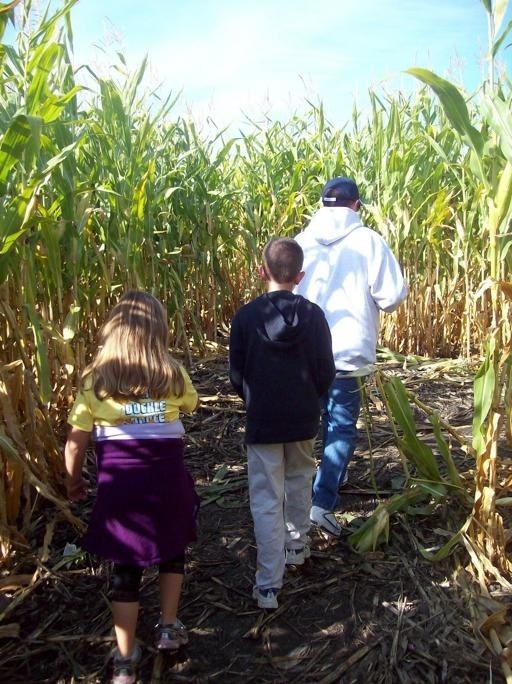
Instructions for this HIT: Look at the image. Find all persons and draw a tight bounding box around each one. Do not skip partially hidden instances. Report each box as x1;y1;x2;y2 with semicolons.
283;174;407;536
64;288;199;683
226;234;335;609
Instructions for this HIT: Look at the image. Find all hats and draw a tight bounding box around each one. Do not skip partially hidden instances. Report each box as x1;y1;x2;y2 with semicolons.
322;177;359;201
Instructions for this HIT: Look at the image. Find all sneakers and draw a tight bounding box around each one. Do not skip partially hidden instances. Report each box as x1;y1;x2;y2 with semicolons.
112;647;142;684
155;620;188;649
253;585;278;608
285;545;310;564
310;506;341;536
